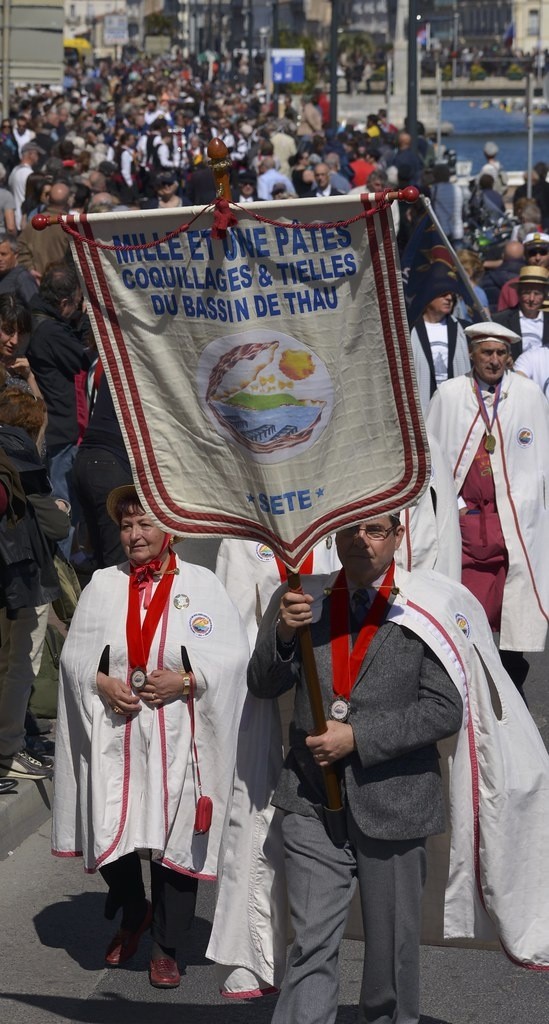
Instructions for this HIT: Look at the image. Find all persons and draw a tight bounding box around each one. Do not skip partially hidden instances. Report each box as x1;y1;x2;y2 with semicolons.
0;30;549;1023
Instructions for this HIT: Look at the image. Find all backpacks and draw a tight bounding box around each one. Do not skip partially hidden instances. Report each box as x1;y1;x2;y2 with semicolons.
27;622;67;719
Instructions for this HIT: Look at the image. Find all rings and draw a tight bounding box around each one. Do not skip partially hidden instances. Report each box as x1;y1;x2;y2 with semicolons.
113;709;119;714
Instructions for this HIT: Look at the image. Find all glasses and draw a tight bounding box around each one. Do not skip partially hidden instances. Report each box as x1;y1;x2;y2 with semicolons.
340;522;399;542
524;249;549;256
162;181;176;187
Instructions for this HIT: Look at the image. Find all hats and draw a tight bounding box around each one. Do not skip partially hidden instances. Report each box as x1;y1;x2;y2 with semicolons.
509;265;549;290
464;321;521;348
21;143;47;155
106;483;185;546
523;232;549;250
160;172;176;183
484;141;498;156
271;183;286;194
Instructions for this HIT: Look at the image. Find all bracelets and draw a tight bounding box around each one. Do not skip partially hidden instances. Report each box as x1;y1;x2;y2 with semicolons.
181;672;190;695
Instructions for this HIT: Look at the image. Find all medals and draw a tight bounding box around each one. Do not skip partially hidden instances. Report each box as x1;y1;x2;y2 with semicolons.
328;697;351;723
130;668;148;691
485;434;496;451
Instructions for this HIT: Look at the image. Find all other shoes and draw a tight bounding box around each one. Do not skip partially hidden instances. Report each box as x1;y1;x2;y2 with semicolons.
25;738;56;755
19;741;53;767
151;941;180;987
0;776;19;794
105;899;153;964
0;751;54;778
23;718;52;735
